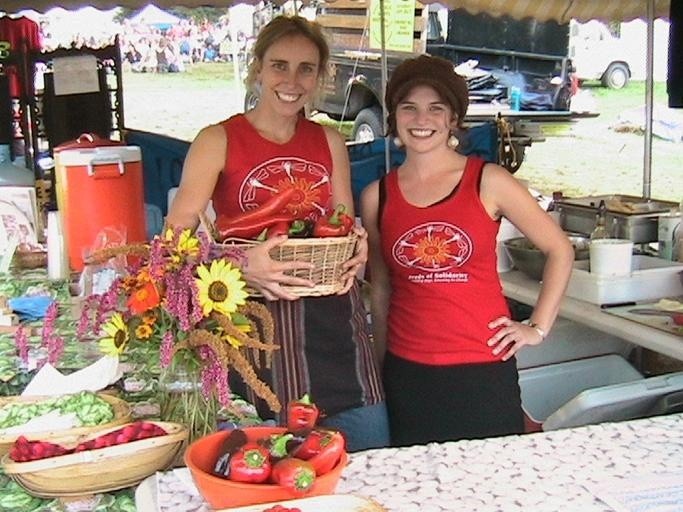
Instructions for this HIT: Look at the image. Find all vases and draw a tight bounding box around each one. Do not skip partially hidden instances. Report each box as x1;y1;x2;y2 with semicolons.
140;345;223;473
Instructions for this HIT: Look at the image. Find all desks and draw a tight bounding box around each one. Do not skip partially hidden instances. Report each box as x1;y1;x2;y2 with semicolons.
131;407;681;512
493;256;682;364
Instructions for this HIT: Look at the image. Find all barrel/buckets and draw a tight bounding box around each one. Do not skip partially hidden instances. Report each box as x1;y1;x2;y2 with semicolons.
146;205;164;237
53;134;145;272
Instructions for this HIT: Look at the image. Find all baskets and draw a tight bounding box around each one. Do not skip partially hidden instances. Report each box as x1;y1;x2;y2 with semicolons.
15;252;47;267
216;232;357;297
0;394;188;498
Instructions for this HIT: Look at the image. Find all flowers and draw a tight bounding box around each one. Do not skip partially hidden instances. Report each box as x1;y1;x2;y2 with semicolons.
6;220;288;468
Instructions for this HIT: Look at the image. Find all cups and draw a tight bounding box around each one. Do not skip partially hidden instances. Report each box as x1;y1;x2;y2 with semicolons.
587;238;634;280
657;214;680;260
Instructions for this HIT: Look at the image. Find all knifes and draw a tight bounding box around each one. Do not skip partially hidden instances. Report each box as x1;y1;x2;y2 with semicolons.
595;296;659;310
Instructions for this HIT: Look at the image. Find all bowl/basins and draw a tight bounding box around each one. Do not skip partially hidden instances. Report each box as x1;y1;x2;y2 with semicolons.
182;426;348;511
502;237;590;282
12;251;47;269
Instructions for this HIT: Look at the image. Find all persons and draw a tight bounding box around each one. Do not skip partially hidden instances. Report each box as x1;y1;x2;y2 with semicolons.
159;16;391;453
358;54;575;448
37;16;258;76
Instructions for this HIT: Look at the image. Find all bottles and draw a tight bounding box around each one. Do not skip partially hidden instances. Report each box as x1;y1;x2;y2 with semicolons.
510;84;520;112
588;199;612;270
546;191;563;214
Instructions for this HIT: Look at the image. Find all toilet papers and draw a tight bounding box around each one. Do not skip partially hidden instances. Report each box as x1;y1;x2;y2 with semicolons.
48;211;71;281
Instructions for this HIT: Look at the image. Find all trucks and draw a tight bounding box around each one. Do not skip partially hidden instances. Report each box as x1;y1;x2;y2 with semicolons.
241;0;602;176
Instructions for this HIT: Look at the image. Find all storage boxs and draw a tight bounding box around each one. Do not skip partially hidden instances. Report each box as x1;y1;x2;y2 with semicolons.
563;254;682;308
514;316;683;435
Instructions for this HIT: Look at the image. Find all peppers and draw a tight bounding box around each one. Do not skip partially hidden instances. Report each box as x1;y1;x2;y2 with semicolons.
212;187;353;242
229;394;344;491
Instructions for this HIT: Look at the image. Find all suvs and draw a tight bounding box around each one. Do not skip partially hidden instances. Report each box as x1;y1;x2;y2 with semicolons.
565;16;638;92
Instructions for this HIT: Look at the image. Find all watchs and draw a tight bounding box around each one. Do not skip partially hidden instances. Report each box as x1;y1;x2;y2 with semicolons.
520;320;546;340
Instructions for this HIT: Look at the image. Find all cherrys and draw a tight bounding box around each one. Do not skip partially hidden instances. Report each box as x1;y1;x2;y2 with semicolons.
9;422;166;462
